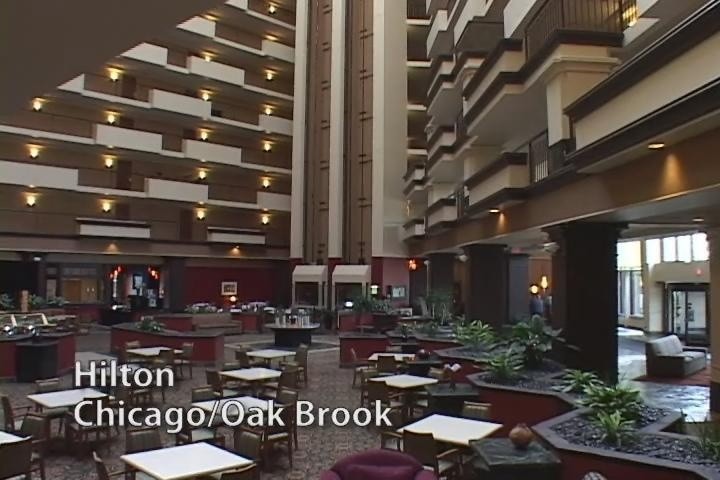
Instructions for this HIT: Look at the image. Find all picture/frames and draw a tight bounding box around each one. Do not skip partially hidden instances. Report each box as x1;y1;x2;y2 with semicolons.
388;285;408;300
218;279;240;297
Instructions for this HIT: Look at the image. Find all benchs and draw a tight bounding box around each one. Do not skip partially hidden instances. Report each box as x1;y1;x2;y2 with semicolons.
644;334;707;378
192;311;243;336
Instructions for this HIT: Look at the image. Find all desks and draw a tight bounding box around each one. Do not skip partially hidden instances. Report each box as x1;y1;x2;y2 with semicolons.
397;412;505;464
263;322;321;346
